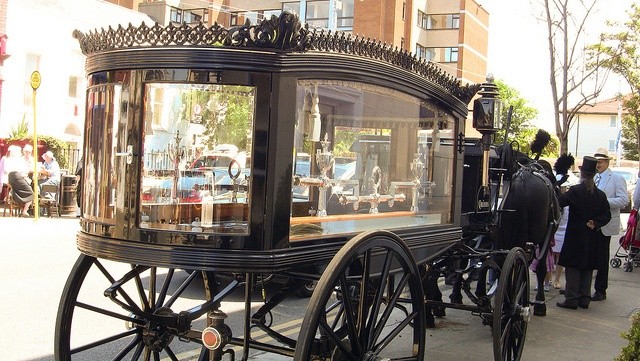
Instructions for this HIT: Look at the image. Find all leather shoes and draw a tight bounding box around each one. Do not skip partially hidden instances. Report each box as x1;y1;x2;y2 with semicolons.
591;290;606;301
579;301;588;309
557;300;578;309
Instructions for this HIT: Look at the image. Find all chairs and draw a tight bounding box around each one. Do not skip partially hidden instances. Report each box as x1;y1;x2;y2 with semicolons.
42;185;58;218
3;185;18;217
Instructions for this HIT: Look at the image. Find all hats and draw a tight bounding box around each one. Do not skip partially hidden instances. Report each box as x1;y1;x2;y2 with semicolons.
593;147;613;160
578;156;597;174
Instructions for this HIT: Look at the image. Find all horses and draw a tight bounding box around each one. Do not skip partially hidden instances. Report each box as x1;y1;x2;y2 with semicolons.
419;145;562;327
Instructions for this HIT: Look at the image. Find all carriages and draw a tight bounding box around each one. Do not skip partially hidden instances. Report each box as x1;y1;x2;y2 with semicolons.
54;9;570;360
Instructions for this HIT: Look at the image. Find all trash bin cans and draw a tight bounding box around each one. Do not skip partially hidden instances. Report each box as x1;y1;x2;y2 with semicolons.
61;175;78;213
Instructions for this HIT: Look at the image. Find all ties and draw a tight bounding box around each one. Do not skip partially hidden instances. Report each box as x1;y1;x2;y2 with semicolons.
595;173;600;187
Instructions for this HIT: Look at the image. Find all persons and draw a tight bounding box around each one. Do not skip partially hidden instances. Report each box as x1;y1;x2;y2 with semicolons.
37;151;61;216
632;170;640;210
0;145;15;203
189;147;202;167
590;147;630;300
21;145;34;185
529;234;557;292
549;174;570;289
74;157;83;217
555;156;612;310
7;146;35;218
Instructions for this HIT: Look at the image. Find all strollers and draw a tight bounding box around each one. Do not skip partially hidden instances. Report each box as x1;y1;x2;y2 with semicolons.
610;204;639;272
